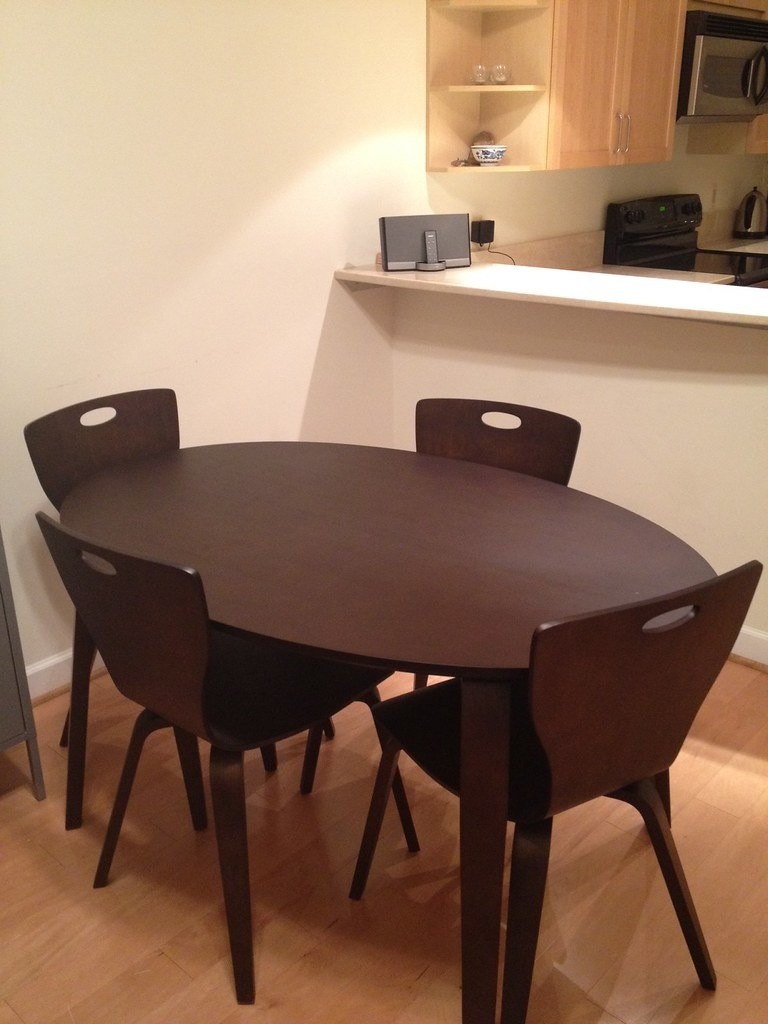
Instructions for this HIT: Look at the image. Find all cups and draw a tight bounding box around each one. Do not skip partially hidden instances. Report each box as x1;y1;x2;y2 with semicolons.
469;63;489;85
490;63;511;85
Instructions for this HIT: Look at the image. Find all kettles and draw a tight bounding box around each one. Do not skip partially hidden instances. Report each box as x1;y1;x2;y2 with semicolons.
733;186;768;239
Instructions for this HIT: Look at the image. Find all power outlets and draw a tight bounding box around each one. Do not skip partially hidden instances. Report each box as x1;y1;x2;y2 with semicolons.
472;214;490;251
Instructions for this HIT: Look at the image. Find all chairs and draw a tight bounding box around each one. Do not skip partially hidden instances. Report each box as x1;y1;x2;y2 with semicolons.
414;398;583;487
34;511;422;1006
347;559;766;1024
25;388;337;834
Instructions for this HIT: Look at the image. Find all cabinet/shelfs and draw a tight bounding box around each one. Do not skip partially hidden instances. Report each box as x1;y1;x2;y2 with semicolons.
685;114;768;154
426;0;688;174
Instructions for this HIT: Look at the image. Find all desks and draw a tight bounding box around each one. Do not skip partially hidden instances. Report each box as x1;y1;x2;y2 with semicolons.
573;264;736;285
698;236;768;274
60;441;719;1024
331;259;768;673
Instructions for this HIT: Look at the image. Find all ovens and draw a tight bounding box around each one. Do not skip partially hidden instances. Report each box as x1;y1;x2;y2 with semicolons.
676;10;768;127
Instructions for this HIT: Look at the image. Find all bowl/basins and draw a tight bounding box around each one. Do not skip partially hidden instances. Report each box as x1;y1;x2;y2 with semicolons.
470;145;507;167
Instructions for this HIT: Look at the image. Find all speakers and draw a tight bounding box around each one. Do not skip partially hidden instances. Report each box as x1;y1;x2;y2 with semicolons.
379;212;472;270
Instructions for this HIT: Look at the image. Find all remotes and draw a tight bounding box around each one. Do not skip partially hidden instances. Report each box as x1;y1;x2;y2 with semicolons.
424;230;438;264
